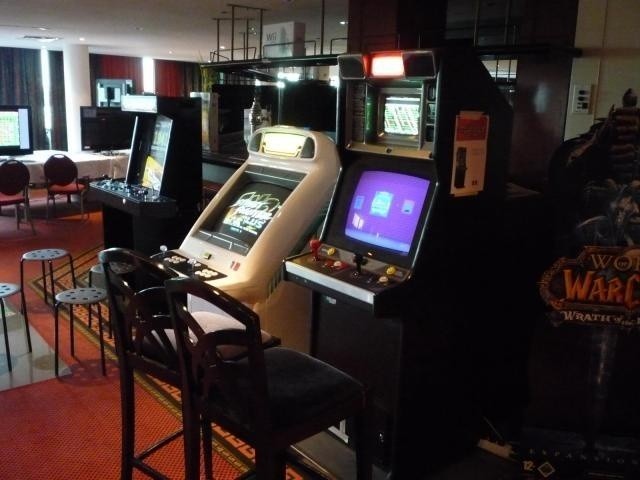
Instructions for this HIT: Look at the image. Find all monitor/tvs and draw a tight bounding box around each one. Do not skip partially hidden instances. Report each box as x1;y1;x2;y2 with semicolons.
96;79;134;106
208;179;298;247
121;95;159;115
0;105;34;157
378;93;421;138
344;168;431;260
79;105;137;151
139;118;176;190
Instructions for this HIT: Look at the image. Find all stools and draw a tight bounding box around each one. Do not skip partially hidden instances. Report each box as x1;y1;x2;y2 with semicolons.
19;246;78;325
0;283;33;375
86;264;115;341
50;288;110;377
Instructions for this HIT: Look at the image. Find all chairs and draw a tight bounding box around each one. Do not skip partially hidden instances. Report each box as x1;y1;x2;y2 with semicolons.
43;154;90;223
166;276;375;479
0;161;37;235
97;247;282;480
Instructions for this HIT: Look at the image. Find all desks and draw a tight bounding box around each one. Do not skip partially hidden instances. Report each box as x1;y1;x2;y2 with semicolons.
98;148;131;180
0;144;112;211
